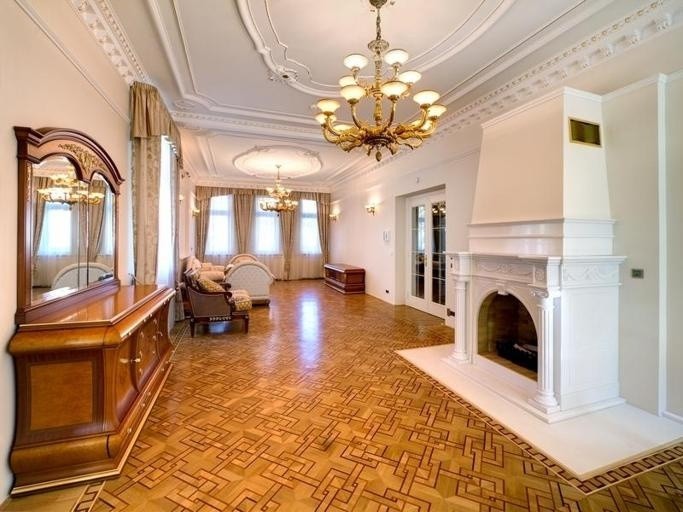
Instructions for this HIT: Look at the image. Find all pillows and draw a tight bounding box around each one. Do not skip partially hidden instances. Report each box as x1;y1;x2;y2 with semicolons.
194;277;226;294
200;262;211;270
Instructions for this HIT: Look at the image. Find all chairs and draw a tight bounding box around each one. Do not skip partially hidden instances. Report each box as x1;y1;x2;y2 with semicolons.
50;258;111;291
224;253;258;273
182;267;252;338
224;259;275;307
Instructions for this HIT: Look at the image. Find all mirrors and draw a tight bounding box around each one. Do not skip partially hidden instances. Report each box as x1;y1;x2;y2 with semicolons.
13;125;126;325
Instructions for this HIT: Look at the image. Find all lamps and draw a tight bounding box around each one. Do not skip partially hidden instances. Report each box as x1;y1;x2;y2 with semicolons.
311;1;449;163
328;214;336;224
364;204;375;216
36;161;105;211
431;201;445;215
259;164;298;217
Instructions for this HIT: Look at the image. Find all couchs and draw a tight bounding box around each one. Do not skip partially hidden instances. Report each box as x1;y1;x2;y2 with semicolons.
185;254;224;281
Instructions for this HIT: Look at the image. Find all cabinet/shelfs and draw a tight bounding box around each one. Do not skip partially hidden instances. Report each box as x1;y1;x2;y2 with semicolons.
4;287;177;496
322;263;365;295
